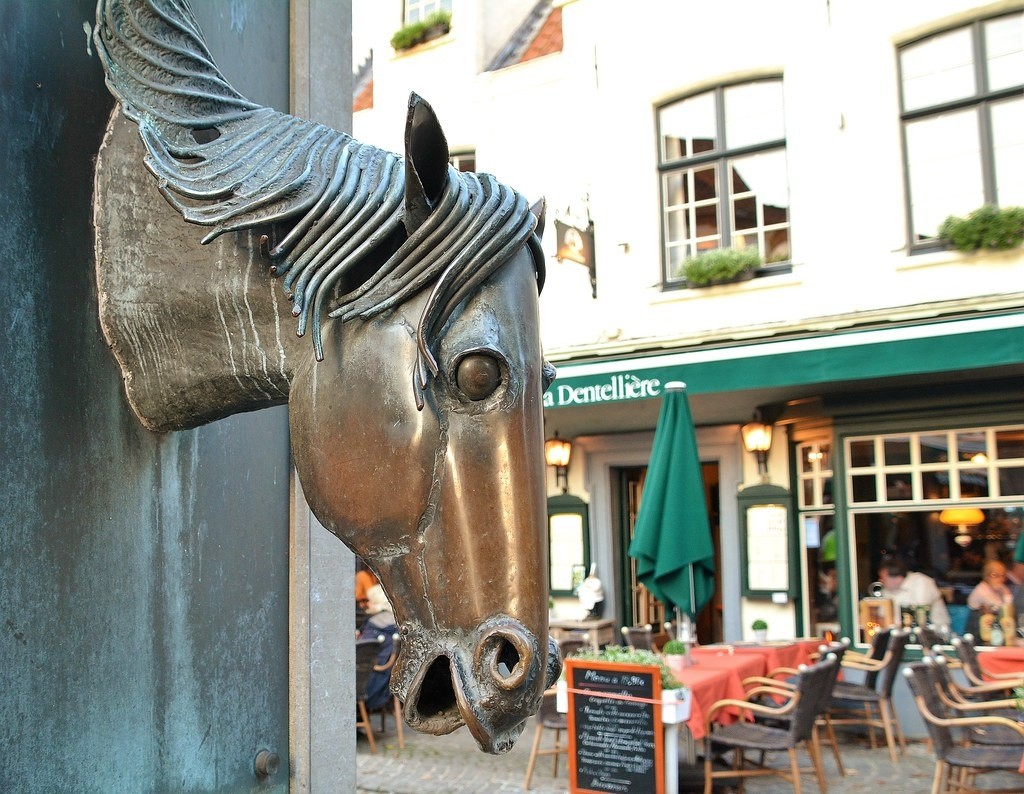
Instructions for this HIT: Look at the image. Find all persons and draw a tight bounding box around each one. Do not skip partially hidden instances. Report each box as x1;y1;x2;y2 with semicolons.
877;559;951;626
966;561;1013;642
816;568;839;623
356;584;399;737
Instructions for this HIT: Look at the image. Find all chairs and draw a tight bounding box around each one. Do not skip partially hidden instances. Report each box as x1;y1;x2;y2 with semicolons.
356;634;404;752
525;622;1024;794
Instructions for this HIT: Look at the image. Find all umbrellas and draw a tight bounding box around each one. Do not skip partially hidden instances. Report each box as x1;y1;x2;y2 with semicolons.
628;381;716;623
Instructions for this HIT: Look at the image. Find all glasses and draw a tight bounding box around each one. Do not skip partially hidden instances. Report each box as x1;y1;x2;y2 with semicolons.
988;573;1007;578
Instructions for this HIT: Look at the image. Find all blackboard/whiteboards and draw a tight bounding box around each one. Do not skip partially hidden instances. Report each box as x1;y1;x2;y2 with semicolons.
563;658;665;794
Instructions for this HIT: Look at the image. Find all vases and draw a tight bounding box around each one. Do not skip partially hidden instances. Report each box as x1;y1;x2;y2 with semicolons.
555;681;693;724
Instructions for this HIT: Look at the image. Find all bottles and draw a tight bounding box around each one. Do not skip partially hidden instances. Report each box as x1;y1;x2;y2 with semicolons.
989;608;1004;647
902;605;930;644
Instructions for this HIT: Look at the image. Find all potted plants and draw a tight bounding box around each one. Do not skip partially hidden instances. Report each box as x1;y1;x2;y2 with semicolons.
936;207;1023;253
391;10;449;51
673;246;758;286
663;641;684;672
752;620;768;644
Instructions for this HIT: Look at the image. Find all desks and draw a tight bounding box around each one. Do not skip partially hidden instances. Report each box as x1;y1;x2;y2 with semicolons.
549;619;615;654
689;638;830;705
979;646;1024;696
663;652;764;738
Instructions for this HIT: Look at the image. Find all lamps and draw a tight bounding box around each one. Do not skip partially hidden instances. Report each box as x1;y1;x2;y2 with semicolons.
938;491;985;548
543;429;572;489
740;407;775;472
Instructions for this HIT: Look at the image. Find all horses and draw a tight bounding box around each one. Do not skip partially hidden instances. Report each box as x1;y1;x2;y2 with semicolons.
91;1;563;760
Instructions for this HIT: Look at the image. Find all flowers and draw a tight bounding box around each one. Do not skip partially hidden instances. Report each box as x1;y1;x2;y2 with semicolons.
562;647;685;690
